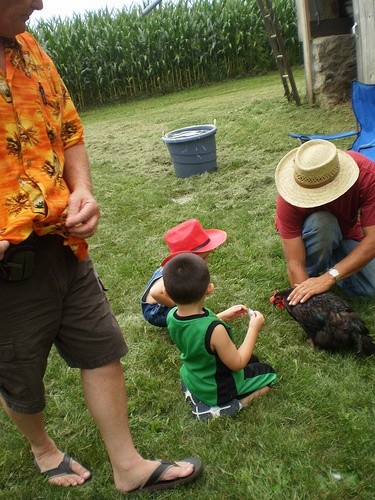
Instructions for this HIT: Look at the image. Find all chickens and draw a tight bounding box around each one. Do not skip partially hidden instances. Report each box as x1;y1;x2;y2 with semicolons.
267;286;375;359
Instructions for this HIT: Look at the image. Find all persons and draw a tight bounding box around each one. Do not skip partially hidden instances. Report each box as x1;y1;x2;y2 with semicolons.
274;139;375;305
142;219;227;328
0;0;203;493
164;253;277;420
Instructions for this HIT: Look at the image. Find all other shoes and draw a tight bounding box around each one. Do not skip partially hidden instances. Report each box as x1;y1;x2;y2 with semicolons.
332;284;354;311
181;379;201;409
191;398;242;421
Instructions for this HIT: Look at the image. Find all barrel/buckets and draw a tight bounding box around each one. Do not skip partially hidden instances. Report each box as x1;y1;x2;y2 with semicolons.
161;119;218;178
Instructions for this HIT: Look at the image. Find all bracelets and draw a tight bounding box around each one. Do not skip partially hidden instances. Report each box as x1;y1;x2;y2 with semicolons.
330;268;339;282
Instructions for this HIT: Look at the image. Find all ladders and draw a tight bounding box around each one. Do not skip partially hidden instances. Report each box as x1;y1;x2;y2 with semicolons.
258;0;301;107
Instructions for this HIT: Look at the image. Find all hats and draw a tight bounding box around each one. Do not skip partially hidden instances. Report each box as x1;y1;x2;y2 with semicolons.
160;218;228;267
275;138;360;208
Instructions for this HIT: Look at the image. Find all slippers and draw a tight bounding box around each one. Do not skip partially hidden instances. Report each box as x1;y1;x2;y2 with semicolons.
127;457;202;493
33;451;94;487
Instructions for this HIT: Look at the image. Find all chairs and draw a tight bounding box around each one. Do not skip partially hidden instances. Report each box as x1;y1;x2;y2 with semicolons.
288;80;375;160
